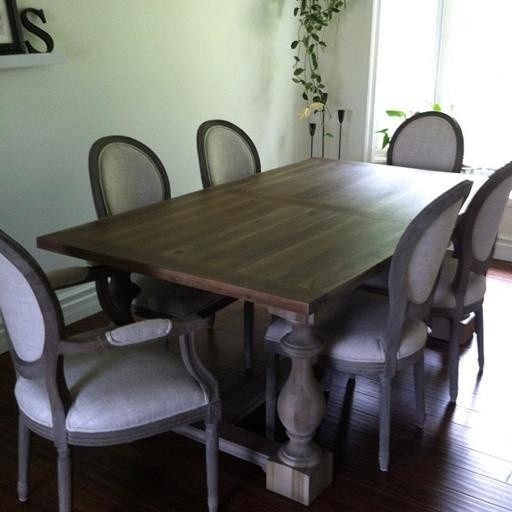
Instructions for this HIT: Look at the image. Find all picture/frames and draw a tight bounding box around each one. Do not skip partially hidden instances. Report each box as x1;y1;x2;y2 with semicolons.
0;0;27;56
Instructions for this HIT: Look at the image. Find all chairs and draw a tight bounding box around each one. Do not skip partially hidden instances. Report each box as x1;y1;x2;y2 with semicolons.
88;135;254;369
0;225;221;512
263;179;473;471
386;111;465;172
196;119;262;189
359;161;512;405
